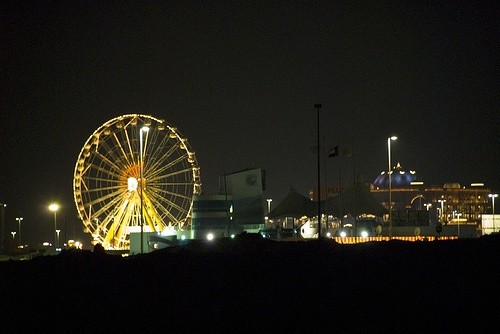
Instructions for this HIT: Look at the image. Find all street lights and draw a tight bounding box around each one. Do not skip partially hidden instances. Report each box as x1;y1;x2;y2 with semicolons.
314;103;323;240
488;193;498;232
455;213;463;237
50;204;58;246
387;136;398;241
438;200;446;222
16;217;23;242
